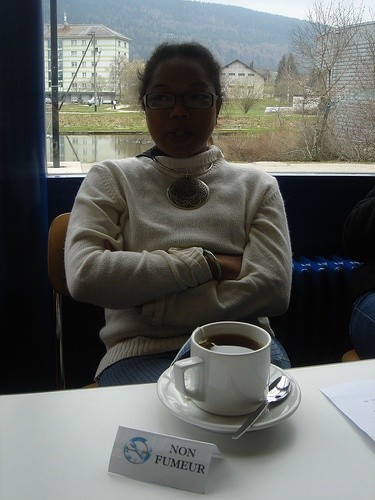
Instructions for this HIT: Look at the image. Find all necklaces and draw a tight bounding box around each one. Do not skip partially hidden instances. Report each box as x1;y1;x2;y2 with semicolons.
151;157;214;210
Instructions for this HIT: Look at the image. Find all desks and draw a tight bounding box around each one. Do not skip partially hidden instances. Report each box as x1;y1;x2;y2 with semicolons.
0;358;375;500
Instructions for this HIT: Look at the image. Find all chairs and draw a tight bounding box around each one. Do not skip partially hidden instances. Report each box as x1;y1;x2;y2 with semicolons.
47;212;98;390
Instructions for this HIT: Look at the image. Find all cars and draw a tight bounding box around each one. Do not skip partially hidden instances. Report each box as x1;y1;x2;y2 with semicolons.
45;97;52;104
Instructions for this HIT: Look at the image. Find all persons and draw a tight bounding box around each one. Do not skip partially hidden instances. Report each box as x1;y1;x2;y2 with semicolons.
64;41;293;388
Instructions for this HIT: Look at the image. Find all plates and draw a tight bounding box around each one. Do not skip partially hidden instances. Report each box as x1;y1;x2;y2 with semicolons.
157;358;301;434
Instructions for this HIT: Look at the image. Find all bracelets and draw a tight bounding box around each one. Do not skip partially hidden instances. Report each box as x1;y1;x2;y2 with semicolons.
203;249;221;281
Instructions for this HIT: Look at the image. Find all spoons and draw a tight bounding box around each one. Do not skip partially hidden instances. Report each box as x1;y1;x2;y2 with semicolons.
231;375;293;440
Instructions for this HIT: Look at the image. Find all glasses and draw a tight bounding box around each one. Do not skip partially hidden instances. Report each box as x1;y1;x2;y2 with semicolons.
142;92;220;111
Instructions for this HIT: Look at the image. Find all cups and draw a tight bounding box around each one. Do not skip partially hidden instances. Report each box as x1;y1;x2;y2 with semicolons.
175;321;271;417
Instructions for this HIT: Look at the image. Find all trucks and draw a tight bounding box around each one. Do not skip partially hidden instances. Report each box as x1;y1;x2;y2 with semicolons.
87;97;117;106
263;96;318;113
